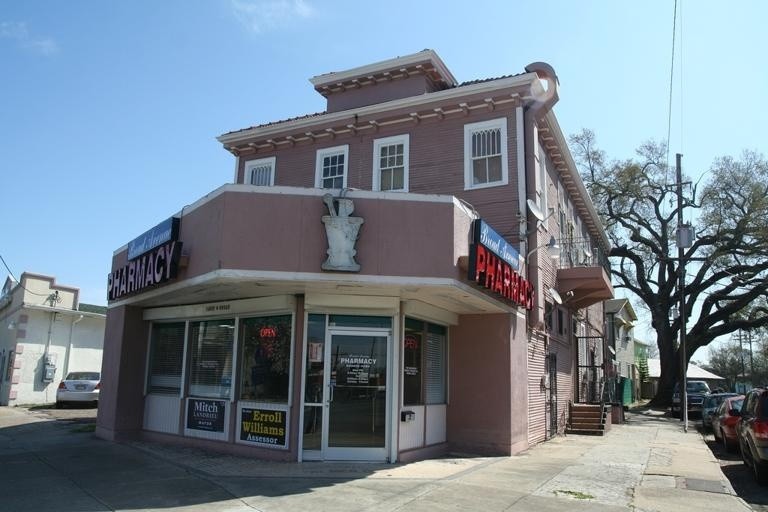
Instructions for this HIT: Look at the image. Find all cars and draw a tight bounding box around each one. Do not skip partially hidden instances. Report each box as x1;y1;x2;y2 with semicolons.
703;389;768;485
672;380;712;418
56;371;101;409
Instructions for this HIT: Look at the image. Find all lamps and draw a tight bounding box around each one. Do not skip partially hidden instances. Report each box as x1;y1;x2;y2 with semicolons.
524;235;560;265
